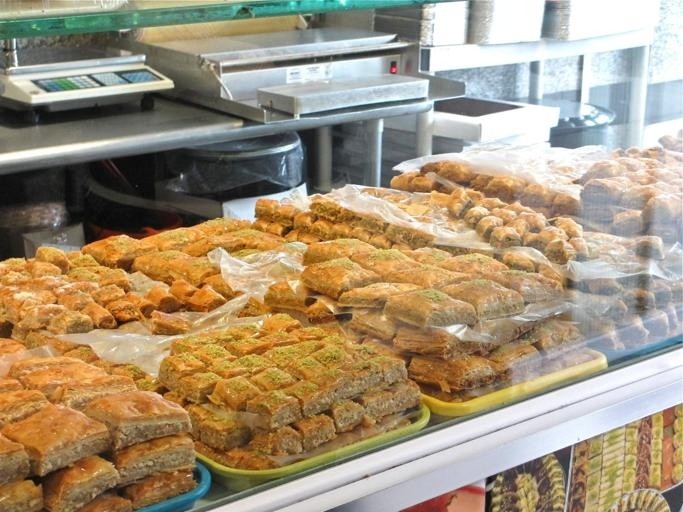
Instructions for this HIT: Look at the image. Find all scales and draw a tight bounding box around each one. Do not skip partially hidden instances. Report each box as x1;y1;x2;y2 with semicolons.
0;46;175;124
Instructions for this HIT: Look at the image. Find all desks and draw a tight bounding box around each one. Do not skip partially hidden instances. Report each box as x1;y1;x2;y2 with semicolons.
0;72;431;189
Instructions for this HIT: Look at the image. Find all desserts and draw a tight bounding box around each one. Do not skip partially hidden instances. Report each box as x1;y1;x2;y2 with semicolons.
0;129;683;512
